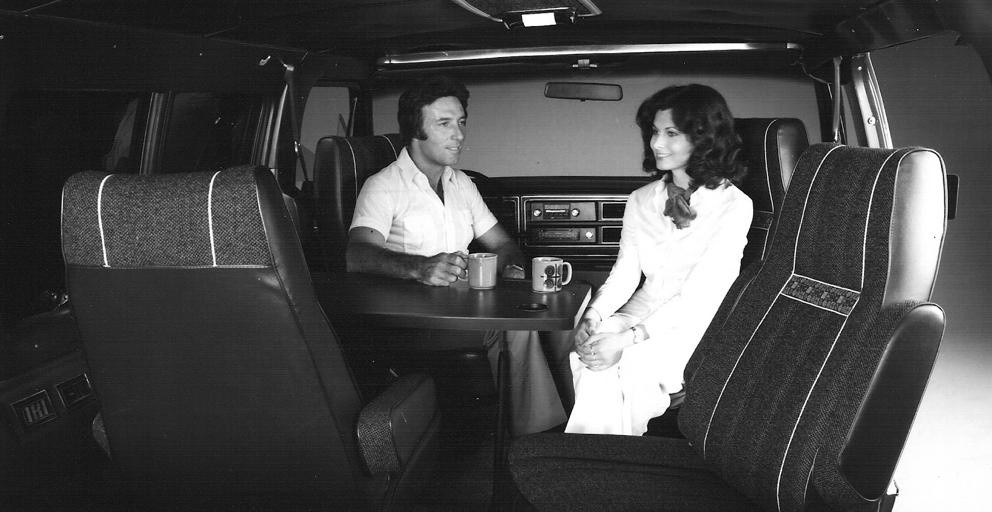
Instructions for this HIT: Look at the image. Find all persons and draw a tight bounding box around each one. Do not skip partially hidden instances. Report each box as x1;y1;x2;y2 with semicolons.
344;76;568;438
565;82;756;436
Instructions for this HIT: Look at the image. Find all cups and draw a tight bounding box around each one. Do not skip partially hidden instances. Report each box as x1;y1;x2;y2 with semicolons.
532;256;573;292
455;253;498;290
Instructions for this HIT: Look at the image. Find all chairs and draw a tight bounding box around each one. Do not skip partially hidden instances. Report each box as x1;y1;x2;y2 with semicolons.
314;132;405;273
496;142;948;512
59;172;442;512
731;116;808;272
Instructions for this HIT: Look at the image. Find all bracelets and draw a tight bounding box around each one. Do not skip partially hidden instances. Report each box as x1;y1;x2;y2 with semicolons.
630;324;642;346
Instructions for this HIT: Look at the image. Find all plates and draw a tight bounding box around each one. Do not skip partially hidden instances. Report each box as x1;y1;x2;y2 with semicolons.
513;302;549;313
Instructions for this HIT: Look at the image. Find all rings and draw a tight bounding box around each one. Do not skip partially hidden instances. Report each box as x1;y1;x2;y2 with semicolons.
589;346;596;355
592;354;597;361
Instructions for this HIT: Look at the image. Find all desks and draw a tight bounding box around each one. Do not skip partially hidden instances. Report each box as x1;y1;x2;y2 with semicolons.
311;272;594;332
